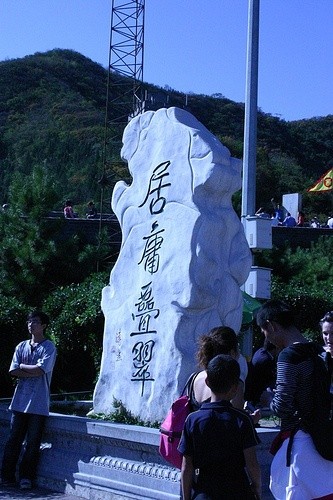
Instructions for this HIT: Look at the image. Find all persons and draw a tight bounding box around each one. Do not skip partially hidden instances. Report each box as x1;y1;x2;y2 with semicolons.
0;311;57;490
85;201;98;219
250;198;333;228
63;199;75;218
177;299;333;500
2;204;10;211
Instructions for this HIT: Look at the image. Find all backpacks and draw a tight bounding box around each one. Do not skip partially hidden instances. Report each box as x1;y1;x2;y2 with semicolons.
292;341;332;461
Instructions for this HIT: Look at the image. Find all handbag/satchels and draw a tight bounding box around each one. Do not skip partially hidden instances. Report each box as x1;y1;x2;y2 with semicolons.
157;369;203;469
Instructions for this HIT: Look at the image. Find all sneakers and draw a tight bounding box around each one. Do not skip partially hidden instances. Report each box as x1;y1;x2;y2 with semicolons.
0;478;11;484
20;479;32;488
